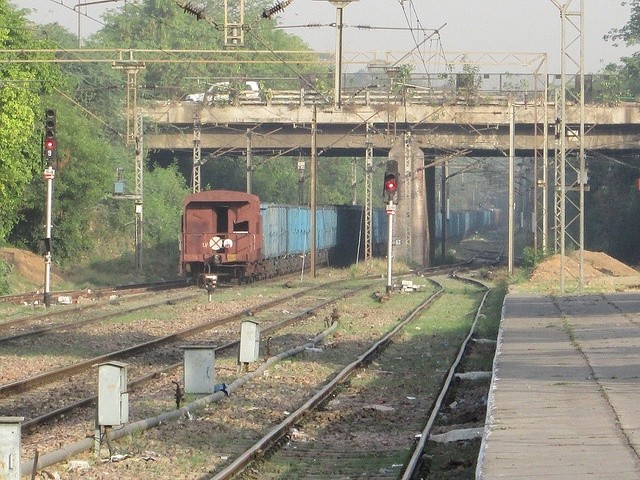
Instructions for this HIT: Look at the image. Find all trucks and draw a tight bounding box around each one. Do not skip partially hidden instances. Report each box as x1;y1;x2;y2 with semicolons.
178;190;503;286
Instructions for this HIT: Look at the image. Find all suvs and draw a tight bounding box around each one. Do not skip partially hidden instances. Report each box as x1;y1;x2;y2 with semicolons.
184;81;261;102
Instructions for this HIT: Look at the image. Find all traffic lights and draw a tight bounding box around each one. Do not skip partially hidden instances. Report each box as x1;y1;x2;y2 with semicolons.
45;108;56;159
383;160;400;205
